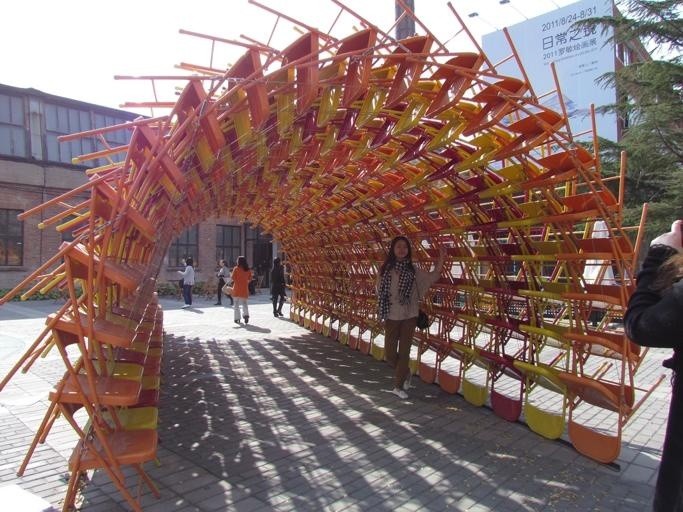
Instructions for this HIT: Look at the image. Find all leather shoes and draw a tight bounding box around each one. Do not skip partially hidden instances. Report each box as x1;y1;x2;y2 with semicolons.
274;311;283;316
215;303;221;305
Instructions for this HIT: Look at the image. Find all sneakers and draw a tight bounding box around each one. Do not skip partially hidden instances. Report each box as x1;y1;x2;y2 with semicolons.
403;368;412;390
393;388;409;399
182;304;190;309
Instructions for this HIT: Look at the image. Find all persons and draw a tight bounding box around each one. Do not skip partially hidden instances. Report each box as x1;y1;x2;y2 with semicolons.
214;259;235;306
621;218;683;512
176;259;194;309
376;236;444;399
269;257;286;317
230;256;252;324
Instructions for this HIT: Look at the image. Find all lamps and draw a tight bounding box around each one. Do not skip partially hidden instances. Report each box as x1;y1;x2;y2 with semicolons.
466;11;500;33
498;0;528;20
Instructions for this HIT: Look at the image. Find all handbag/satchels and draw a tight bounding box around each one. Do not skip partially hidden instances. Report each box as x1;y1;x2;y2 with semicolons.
221;284;233;295
417;309;428;329
180;276;195;288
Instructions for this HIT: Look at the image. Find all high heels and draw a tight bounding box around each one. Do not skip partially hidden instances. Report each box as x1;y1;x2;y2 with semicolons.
235;319;240;323
